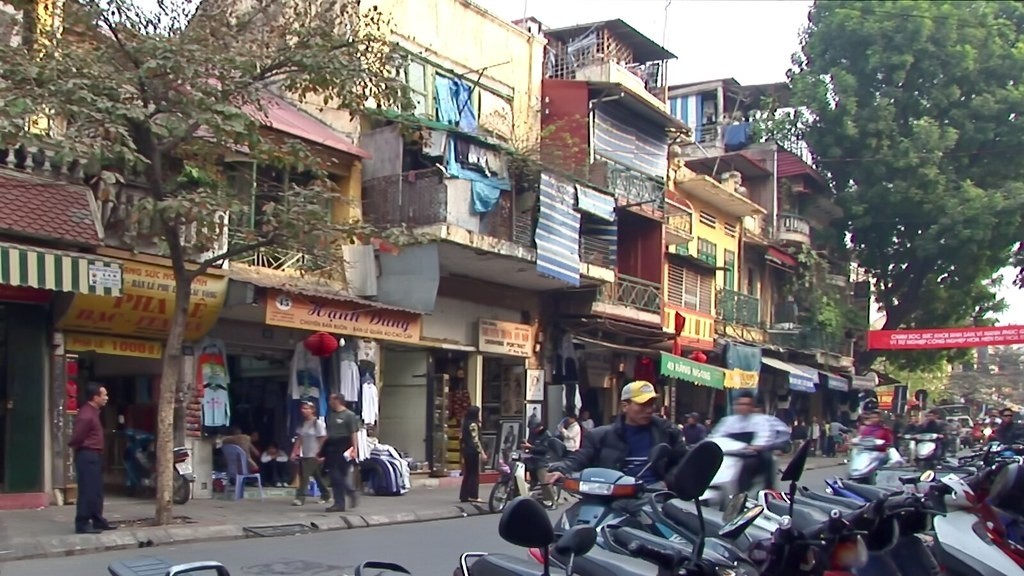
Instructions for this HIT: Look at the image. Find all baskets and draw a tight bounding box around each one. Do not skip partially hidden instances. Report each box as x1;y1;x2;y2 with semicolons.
495;460;517;478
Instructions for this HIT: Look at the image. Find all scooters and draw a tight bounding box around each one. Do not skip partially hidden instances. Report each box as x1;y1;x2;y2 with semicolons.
691;436;758;511
844;434;889;485
546;437;672;534
912;433;943;471
488;449;560;515
107;553;230;576
454;441;1024;576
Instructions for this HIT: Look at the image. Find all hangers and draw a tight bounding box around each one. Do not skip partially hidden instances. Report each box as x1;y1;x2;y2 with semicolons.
342;337;376;385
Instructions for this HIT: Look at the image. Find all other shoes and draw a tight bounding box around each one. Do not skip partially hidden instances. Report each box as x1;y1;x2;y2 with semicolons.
316;498;333;503
460;497;472;502
95;523;117;530
548;504;557;510
292;499;302;506
326;505;345;512
76;527;101;534
276;482;282;486
350;495;357;508
469;497;483;502
528;547;552;565
283;482;288;487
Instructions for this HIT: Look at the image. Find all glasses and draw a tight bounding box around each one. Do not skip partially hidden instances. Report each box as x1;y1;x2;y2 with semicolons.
1003;414;1013;417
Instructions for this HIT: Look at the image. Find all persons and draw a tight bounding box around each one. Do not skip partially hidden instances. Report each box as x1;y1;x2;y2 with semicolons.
547;381;688;486
653;406;714;445
459;405;488;503
234;394;359;512
501;425;515;465
528;406;541;435
520;409;595;491
861;408;1024;470
698;392;792;492
789;415;853;458
68;380;118;534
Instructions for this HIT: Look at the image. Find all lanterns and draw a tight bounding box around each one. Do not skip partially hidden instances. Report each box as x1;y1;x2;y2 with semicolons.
687;350;707;363
304;331;339;357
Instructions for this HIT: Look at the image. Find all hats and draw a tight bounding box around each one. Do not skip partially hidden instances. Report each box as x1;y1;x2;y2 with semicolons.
811;416;818;421
685;412;698;419
621;380;660;404
528;417;540;428
567;413;576;418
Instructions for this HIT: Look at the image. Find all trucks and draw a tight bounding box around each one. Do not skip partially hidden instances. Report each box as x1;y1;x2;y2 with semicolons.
936;404;975;447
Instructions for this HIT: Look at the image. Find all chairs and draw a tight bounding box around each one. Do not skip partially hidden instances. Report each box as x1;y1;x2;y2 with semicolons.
223;444;267;499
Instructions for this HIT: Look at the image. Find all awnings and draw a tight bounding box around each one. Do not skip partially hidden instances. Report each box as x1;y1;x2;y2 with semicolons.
723;81;808;111
761;357;875;392
573;335;724;390
588;81;691;133
1;242;124;297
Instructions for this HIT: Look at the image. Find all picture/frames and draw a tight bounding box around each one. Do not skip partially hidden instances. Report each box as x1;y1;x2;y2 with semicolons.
483;368;547;469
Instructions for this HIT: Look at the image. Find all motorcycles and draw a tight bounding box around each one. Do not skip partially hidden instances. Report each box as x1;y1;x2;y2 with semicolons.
112;415;196;504
